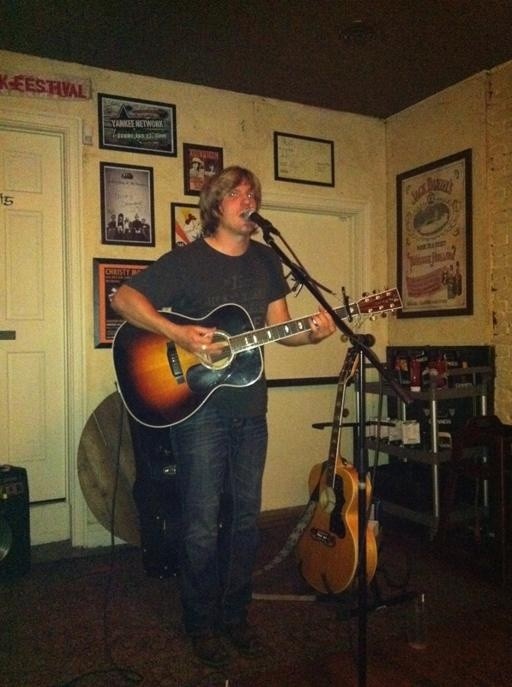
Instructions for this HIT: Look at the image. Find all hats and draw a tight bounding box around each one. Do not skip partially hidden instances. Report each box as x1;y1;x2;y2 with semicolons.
191;157;204;167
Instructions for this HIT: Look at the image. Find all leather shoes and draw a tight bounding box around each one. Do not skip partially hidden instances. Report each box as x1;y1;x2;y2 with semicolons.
191;624;230;666
228;621;269;659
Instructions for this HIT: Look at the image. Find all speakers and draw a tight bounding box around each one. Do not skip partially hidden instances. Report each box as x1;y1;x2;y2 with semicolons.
0;463;33;573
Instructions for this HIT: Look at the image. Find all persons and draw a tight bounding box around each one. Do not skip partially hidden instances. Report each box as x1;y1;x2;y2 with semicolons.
191;159;217;181
178;214;204;248
110;165;337;667
109;212;150;240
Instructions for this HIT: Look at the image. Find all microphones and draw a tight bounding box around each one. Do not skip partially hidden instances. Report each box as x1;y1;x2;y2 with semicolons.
240;204;283;239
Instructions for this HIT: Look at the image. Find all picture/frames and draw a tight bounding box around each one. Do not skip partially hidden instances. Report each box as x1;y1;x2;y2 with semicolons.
183;142;223;196
170;202;203;250
274;132;334;187
395;148;473;319
100;161;155;247
98;92;176;157
93;258;156;349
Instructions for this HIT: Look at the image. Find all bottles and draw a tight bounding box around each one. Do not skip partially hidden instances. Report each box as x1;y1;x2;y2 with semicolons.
434;355;446;375
407;576;427;647
410;356;422;392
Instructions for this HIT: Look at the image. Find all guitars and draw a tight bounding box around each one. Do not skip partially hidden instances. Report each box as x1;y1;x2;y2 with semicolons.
113;286;404;430
295;334;377;596
